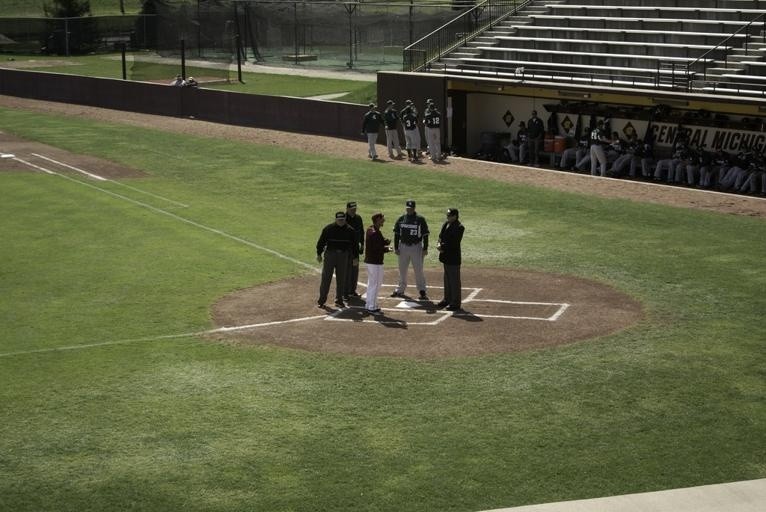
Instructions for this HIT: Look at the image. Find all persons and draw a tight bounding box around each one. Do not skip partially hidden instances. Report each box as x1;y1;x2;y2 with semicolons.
360;98;446;167
344;200;364;301
314;211;358;309
389;199;431;300
365;211;394;316
436;207;466;310
505;109;766;196
172;74;186;86
184;76;198;87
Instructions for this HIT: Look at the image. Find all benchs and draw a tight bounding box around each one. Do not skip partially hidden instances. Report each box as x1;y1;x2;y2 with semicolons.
419;0;766;99
502;139;766;196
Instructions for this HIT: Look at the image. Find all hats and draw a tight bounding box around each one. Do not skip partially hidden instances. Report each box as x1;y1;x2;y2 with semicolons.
447;208;458;216
369;99;436;113
347;202;357;209
336;212;346;220
406;200;415;209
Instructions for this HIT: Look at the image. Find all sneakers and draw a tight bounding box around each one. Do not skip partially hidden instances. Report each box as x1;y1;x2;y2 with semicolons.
333;293;361;308
438;301;460;309
419;290;428;299
390;291;404;296
317;302;323;307
364;309;384;315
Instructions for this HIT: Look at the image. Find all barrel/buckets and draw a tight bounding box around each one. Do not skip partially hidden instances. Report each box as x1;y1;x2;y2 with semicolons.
544;139;554;152
554;135;566;153
